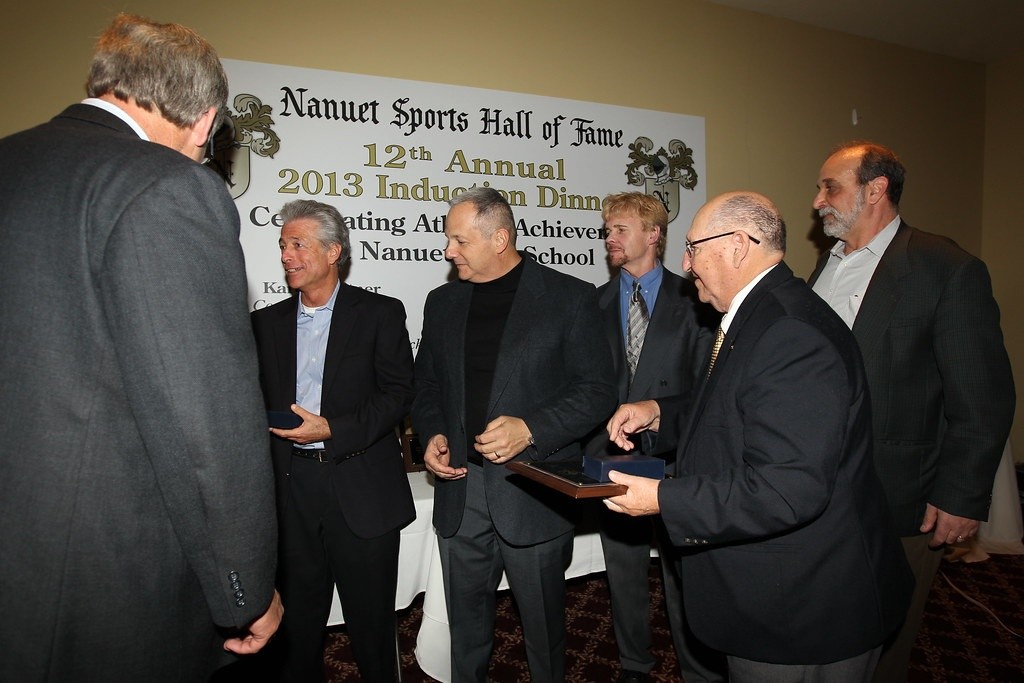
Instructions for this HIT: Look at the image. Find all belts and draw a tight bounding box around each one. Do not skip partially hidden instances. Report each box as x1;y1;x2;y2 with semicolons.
467;456;484;468
292;447;329;463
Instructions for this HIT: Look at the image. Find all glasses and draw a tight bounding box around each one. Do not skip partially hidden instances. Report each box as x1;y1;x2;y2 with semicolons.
686;232;760;258
200;135;215;166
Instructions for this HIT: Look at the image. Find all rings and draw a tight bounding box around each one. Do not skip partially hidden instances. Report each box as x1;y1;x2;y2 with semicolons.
494;451;500;458
956;536;965;544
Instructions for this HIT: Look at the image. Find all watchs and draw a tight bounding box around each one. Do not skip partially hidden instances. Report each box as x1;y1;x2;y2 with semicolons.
527;435;536;446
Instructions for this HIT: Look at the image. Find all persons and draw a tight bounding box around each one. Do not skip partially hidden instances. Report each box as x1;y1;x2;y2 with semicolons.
407;187;619;683
807;142;1016;683
606;190;917;683
248;200;417;683
0;10;285;683
584;192;727;683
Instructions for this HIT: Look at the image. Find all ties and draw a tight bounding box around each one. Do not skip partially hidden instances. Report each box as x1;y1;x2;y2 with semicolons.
706;324;725;383
627;282;649;384
